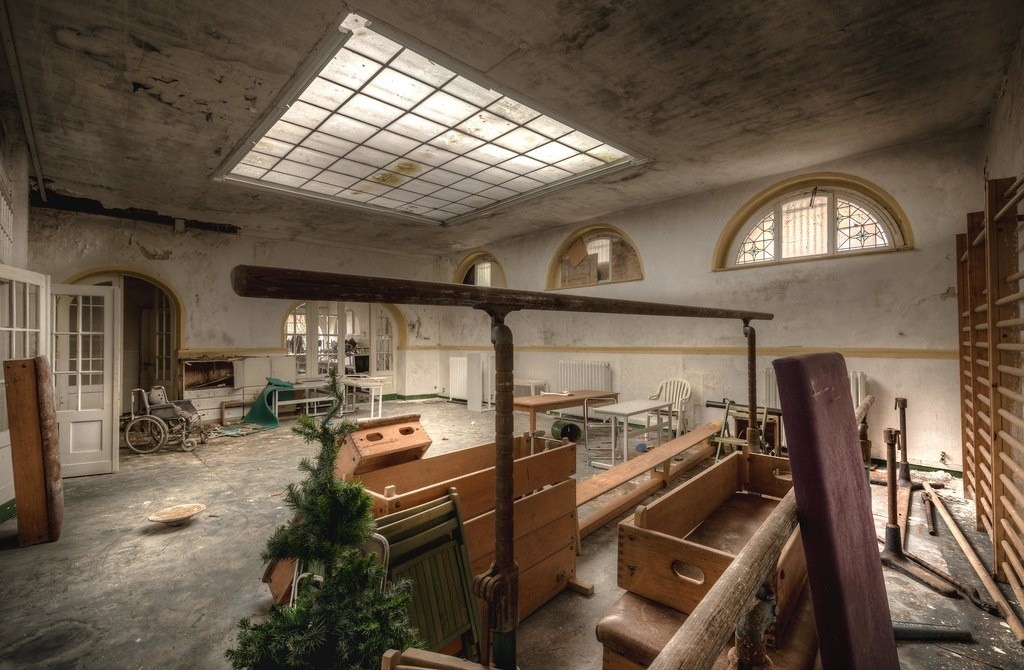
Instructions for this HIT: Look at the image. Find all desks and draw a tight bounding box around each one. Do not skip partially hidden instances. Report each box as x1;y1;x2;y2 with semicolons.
588;401;675;469
513;379;550;415
342;380;393;418
596;493;820;670
271;382;336;421
514;390;620;435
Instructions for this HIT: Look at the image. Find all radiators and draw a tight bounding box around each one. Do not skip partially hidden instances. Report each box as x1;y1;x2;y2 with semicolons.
558;360;611;423
449;356;468;402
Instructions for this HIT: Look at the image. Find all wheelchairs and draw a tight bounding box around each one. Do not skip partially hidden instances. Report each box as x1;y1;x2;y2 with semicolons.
124;385;208;455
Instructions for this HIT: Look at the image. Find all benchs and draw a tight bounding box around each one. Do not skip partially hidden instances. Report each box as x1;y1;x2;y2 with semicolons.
576;422;724;555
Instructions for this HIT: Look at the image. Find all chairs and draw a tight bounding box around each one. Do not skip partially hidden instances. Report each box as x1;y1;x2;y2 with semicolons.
645;378;691;442
585;397;622;459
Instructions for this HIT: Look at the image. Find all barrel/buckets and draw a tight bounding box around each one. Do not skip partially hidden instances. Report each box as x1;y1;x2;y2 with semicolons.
551;420;582;445
